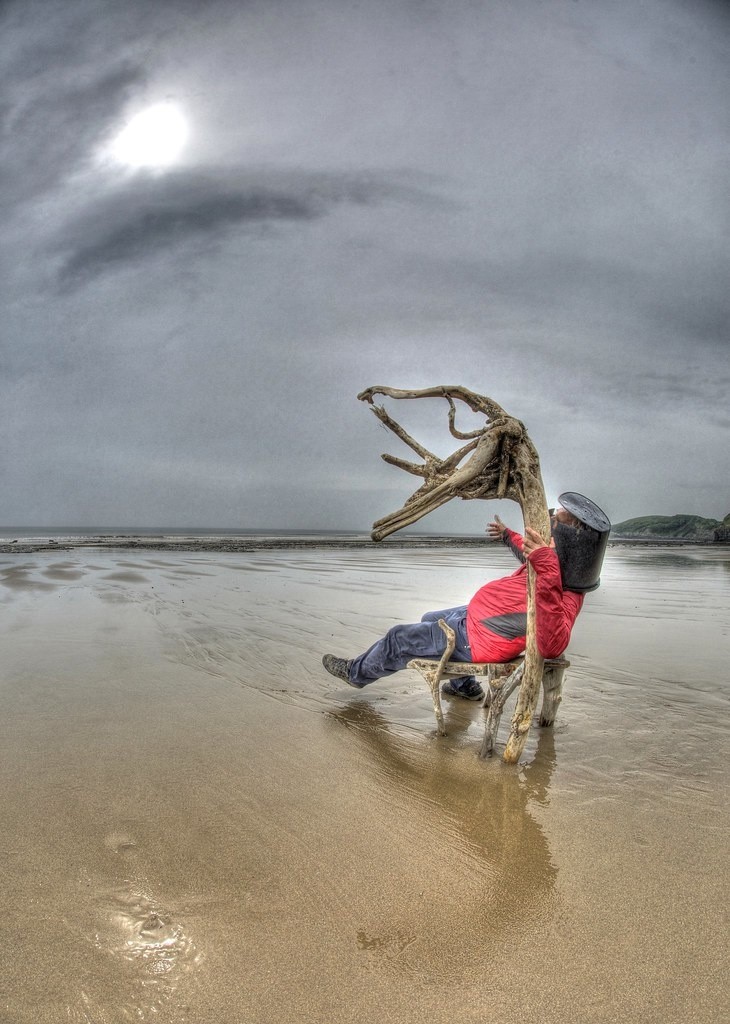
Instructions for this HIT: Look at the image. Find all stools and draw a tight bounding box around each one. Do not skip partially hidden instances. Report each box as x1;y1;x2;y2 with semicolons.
405;653;571;763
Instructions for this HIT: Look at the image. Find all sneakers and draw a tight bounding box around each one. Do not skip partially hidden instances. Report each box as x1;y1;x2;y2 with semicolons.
442;682;484;701
322;654;365;688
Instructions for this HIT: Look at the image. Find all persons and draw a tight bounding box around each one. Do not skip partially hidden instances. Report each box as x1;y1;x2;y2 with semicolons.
322;508;592;701
